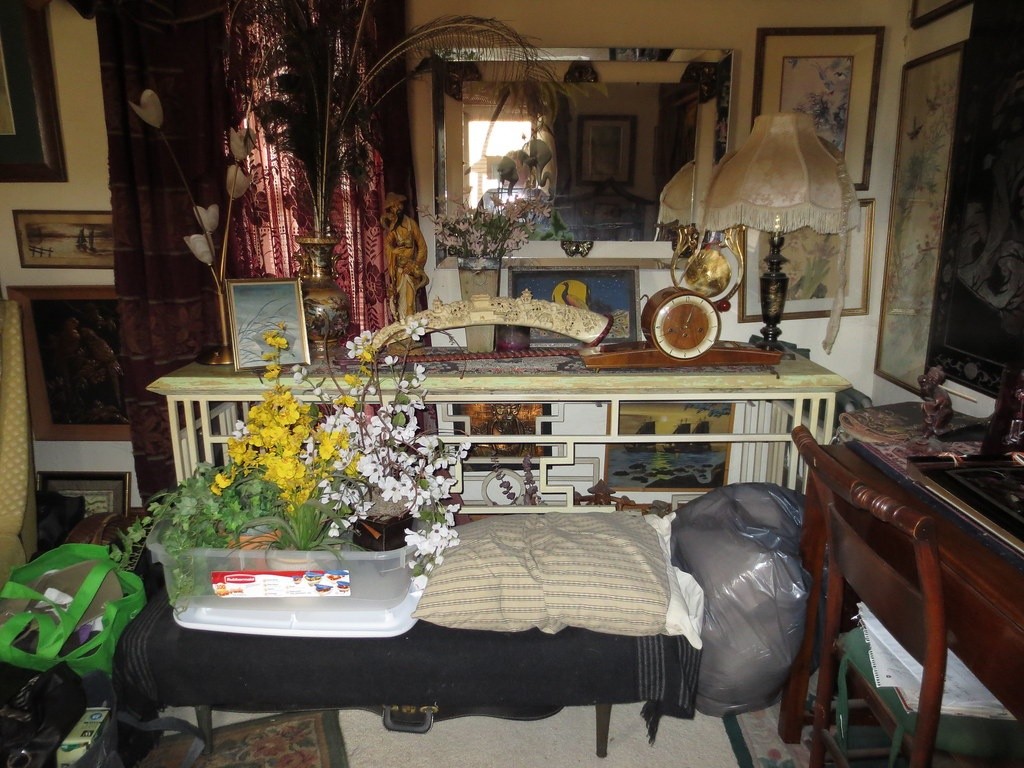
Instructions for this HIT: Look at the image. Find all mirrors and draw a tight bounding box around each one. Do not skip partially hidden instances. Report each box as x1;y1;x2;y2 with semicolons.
461;80;700;242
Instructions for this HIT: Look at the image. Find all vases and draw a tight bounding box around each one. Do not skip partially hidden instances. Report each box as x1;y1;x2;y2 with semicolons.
459;265;502;355
143;520;431;638
208;289;236;369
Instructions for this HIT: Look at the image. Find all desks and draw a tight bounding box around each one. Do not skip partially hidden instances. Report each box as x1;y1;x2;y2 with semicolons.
114;571;703;768
779;415;1024;744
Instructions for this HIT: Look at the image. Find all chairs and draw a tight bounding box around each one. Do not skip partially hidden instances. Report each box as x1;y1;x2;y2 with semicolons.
788;425;1024;768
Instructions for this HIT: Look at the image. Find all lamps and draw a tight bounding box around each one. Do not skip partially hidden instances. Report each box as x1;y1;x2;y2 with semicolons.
699;111;864;353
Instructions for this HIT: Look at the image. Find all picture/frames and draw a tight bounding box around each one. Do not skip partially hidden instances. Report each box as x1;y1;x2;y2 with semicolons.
14;208;117;272
5;283;135;444
226;273;314;375
924;1;1024;404
224;278;313;374
506;263;642;350
36;469;130;518
911;1;968;28
574;113;636;186
873;40;964;394
733;199;871;324
752;23;886;191
0;0;70;188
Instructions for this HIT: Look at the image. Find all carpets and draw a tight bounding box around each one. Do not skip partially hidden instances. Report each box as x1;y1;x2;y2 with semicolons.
139;701;350;768
722;685;841;767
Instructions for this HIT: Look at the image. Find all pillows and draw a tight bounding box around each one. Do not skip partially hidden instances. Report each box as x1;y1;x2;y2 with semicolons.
421;513;670;637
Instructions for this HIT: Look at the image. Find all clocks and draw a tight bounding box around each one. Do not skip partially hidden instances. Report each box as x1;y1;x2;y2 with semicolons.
581;287;782;370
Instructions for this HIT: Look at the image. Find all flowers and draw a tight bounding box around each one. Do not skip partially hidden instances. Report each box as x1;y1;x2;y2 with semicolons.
414;186;556;260
106;314;474;606
129;87;257;281
144;344;852;515
221;1;573;342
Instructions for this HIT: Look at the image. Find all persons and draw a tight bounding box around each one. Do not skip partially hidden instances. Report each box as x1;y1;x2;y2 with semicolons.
475;152;553;229
384;193;427;315
